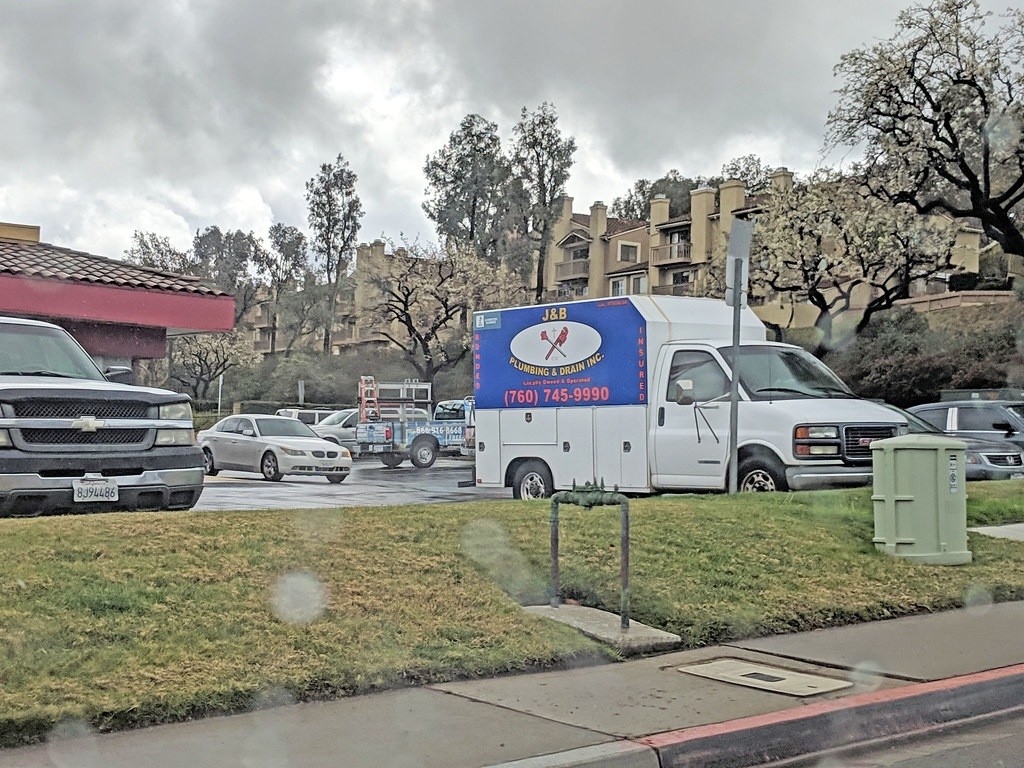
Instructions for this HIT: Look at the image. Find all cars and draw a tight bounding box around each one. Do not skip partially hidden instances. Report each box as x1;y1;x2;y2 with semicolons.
195;414;352;483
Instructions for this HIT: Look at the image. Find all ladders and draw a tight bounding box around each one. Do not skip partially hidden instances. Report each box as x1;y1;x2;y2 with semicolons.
360;375;381;422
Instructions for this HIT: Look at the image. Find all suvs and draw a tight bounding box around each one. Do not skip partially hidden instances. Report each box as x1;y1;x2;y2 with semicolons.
310;406;429;453
903;399;1024;451
0;314;206;517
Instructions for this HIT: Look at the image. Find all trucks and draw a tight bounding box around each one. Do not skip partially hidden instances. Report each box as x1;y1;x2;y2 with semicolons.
354;376;474;468
473;295;907;502
